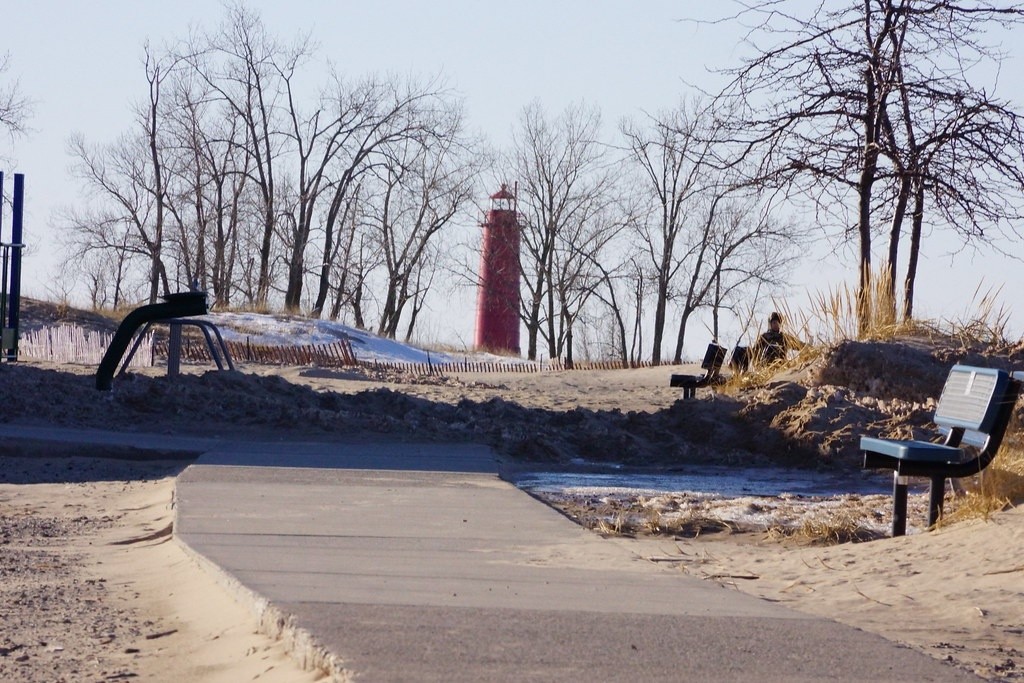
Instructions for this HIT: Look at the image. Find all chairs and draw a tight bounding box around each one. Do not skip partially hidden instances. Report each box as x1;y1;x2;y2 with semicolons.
668;341;787;403
861;363;1020;539
